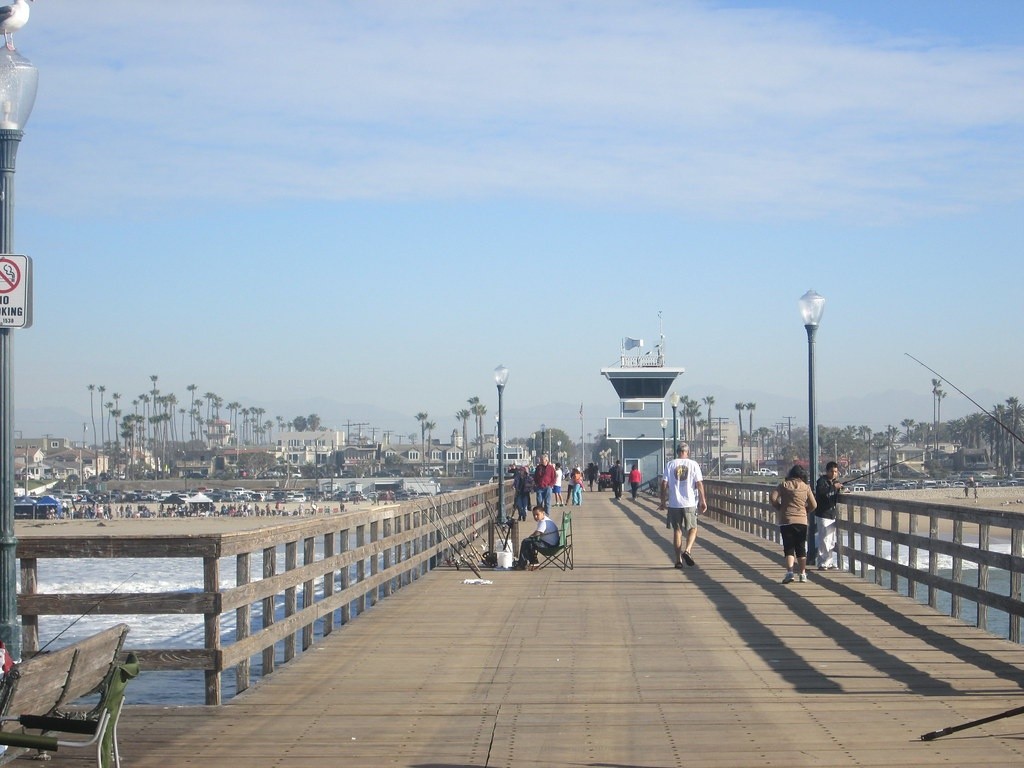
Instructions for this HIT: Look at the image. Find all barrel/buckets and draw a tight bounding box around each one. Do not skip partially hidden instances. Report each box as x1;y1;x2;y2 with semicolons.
495;539;513;553
497;552;512;567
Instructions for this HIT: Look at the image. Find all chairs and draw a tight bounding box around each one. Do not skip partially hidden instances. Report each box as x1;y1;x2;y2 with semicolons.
526;511;574;572
0;652;140;768
305;505;338;515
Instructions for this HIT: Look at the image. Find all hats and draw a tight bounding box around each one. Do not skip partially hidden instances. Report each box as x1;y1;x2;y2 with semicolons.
517;467;526;472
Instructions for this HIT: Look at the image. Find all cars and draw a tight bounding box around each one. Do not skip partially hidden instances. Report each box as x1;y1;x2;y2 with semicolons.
854;471;1024;488
42;470;208;480
13;487;377;502
15;474;29;480
215;468;468;477
722;468;741;475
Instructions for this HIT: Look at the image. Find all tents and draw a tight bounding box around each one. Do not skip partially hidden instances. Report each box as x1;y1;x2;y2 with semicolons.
161;494;184;518
14;495;36;519
36;496;62;519
187;492;213;516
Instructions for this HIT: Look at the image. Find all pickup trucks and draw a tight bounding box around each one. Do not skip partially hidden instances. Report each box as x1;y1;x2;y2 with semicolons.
752;468;778;477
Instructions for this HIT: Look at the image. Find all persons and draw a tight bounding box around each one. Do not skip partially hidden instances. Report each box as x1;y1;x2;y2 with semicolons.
973;487;979;499
339;502;345;512
565;468;586;506
659;442;707;568
609;460;625;501
511;506;560;571
770;464;817;584
962;485;970;499
514;466;535;521
816;462;843;571
628;465;641;501
371;493;380;506
534;454;556;518
552;462;564;507
352;495;360;505
46;500;318;519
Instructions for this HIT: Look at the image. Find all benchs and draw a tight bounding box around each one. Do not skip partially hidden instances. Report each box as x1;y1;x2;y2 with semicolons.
0;623;130;761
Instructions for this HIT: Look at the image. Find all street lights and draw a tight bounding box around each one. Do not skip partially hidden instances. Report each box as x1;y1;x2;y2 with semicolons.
531;433;536;465
0;48;39;679
661;418;668;473
540;424;546;451
494;364;511;528
798;290;827;566
670;391;680;457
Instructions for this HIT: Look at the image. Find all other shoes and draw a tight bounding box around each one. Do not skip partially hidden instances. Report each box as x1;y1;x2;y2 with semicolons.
782;572;794;584
518;515;525;521
682;551;694;565
800;574;806;582
675;562;683;569
553;503;562;507
510;561;540;571
819;563;838;571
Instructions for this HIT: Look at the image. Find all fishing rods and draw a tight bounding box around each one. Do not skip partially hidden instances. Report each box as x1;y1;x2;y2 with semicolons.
905;351;1023;444
656;455;728;511
389;431;513;580
842;448;941;485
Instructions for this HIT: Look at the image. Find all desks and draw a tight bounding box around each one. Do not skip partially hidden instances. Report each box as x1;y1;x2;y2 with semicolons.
36;514;46;519
16;513;32;520
